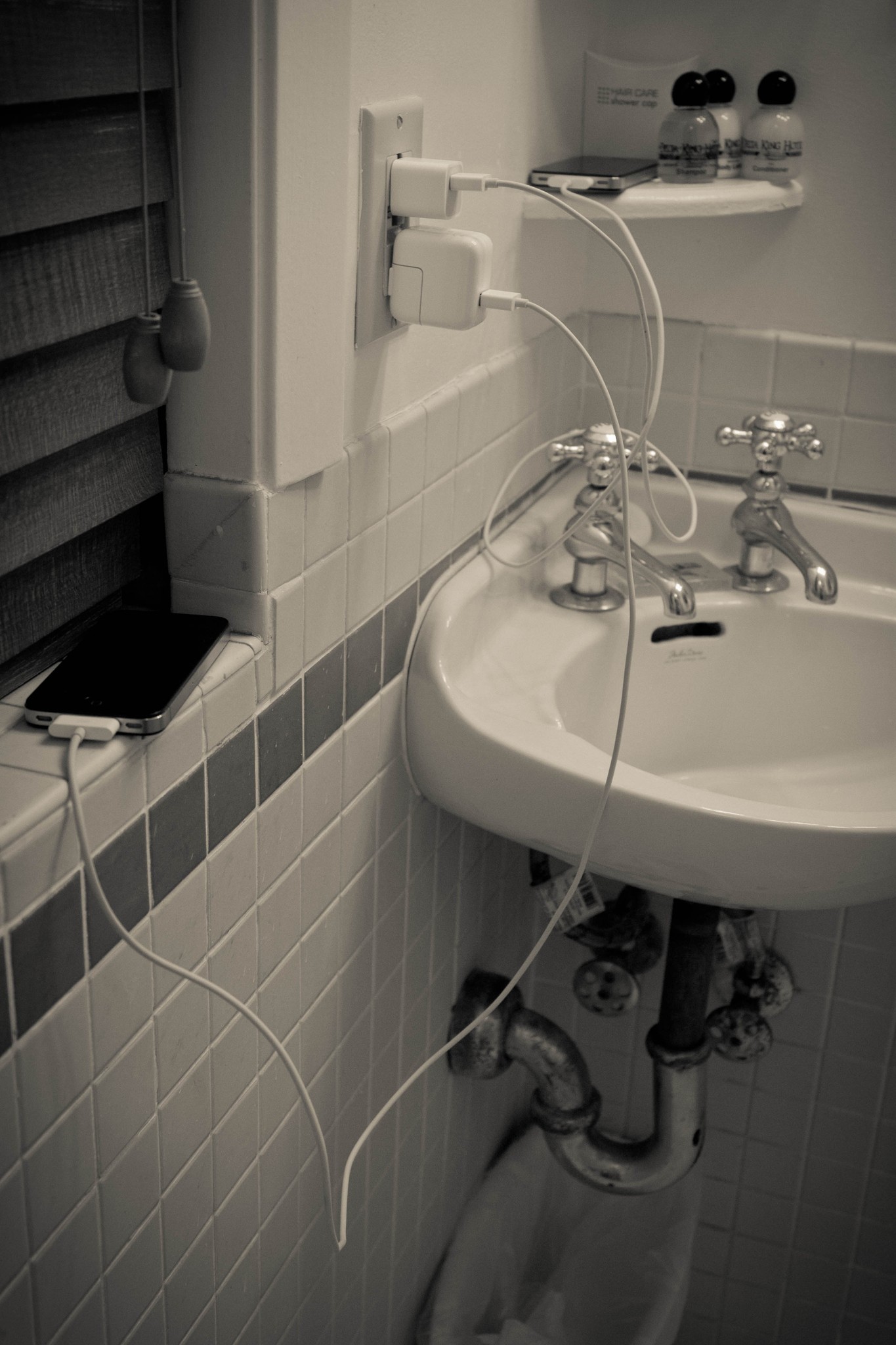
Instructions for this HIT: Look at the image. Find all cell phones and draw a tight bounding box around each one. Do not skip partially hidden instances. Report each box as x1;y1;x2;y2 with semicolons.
529;153;661;195
21;608;232;738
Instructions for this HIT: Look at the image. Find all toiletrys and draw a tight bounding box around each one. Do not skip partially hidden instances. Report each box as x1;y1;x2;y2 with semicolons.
743;69;803;182
657;72;720;183
702;68;742;178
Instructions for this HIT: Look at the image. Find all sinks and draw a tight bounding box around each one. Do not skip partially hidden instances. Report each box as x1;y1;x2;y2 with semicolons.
406;459;896;911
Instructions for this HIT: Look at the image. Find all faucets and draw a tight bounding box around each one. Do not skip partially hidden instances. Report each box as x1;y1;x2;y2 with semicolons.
715;410;839;606
546;422;697;621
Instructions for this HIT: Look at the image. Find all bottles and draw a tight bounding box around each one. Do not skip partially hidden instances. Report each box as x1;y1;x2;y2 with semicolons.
657;70;721;185
740;70;806;181
705;68;743;180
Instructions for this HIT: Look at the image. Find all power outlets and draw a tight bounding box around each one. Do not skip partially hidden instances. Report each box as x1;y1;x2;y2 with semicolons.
350;92;422;352
